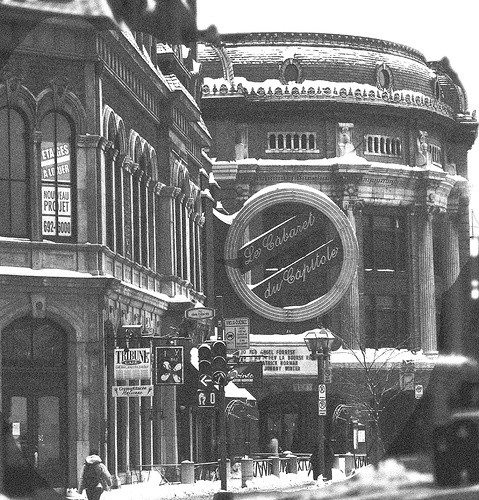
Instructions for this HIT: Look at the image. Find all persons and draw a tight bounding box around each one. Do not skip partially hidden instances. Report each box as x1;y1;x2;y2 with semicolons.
266;437;279;457
77;448;112;500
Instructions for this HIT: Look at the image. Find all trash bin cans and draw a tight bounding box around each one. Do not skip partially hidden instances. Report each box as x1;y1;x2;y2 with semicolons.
339;455;355;477
181;461;195;484
241;458;252;487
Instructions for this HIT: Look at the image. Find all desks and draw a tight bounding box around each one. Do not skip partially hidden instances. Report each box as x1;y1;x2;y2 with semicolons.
279;458;291;474
298;457;312;472
194;461;217;481
133;463;180;487
252;459;273;479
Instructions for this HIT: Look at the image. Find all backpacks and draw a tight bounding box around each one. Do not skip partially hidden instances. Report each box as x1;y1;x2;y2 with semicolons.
81;466;101;488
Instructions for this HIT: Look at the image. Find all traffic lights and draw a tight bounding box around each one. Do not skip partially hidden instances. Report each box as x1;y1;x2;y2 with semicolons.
196;339;227;385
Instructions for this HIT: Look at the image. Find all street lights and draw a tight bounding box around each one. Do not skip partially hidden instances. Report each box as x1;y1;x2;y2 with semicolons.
303;329;336;480
